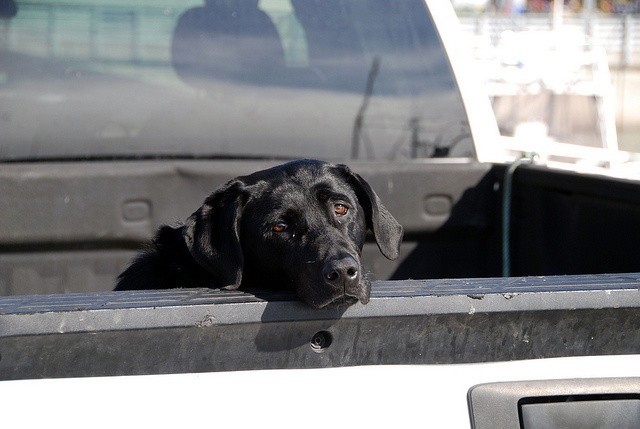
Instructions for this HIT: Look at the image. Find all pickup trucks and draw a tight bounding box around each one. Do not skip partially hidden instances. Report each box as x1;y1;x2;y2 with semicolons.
0;0;640;429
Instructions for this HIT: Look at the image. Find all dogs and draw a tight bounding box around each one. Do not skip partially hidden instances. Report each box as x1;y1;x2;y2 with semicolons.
111;158;404;313
